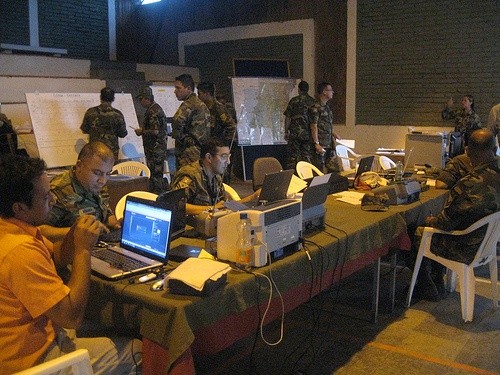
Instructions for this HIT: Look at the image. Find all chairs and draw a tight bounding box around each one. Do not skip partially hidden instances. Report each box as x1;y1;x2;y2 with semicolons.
115;190;159;220
401;211;500;322
110;161;150;178
223;139;396;210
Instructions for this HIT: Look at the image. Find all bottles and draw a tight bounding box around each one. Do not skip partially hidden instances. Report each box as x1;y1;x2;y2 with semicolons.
235;212;252;273
395;156;403;181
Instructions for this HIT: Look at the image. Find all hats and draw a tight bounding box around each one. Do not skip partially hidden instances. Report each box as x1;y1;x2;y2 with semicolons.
136;87;152;99
361;192;389;211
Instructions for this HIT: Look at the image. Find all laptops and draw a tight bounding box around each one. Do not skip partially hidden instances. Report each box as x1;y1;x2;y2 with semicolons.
257;170;295;204
90;188;187;281
345;156;375;181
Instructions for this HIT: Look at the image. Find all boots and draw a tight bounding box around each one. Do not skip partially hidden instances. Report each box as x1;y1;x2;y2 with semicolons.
431;271;444;294
403;269;438;299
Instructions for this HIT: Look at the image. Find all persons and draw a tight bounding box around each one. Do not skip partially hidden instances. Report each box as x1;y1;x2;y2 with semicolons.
78;87;128;167
407;130;500;301
281;81;315;170
136;74;210;195
46;142;121;232
435;94;500;188
167;137;261;214
310;82;341;175
194;81;236;158
136;86;168;194
0;149;142;375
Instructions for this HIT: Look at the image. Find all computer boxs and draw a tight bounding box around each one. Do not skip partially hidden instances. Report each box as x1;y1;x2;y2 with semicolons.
406;133;447;172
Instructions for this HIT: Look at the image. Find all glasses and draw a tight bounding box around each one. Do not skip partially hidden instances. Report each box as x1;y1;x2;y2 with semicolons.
327;89;334;92
215;154;232;159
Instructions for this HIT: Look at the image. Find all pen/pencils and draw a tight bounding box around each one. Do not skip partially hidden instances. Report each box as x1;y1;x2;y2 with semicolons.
129;126;136;130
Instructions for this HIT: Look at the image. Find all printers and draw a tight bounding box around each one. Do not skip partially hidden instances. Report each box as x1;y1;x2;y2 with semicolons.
215;183;332;268
373;178;422;205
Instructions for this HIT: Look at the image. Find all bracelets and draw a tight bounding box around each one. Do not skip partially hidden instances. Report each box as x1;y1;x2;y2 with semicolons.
313;142;319;144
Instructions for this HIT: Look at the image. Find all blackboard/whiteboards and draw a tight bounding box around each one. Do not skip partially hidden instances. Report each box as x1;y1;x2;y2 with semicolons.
24;90;146;171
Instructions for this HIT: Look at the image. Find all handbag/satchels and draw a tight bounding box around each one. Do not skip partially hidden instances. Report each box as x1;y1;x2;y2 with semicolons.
373;177;421;206
354;171;390;190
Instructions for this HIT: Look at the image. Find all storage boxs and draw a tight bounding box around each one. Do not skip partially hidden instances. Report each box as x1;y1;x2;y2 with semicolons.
404;130;451;169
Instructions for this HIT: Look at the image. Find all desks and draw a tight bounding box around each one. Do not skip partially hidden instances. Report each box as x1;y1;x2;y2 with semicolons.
67;197;413;375
342;163;460;314
106;172;150;211
366;150;405;165
